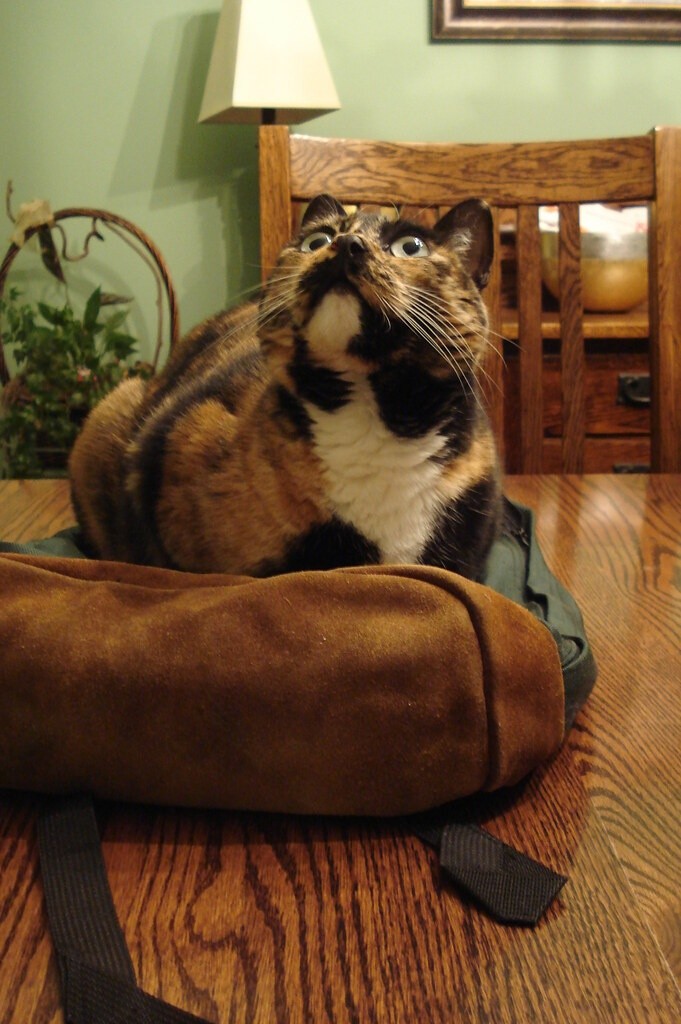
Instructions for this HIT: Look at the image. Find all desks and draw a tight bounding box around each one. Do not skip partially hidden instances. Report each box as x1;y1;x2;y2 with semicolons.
484;306;658;472
1;476;681;1024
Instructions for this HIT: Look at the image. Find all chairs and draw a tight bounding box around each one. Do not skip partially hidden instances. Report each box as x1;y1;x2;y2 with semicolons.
259;126;681;476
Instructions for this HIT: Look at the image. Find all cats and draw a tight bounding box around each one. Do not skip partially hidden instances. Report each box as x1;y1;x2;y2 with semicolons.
66;191;503;587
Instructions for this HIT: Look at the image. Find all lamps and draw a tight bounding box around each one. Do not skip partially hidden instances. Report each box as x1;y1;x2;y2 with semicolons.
198;0;341;134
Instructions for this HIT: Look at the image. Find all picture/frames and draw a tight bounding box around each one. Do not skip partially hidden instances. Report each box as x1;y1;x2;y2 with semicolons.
429;0;681;43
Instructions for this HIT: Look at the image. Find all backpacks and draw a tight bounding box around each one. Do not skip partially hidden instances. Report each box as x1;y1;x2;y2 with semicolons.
0;490;597;817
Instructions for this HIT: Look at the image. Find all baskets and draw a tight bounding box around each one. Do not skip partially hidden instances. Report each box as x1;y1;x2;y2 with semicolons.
1;208;181;465
